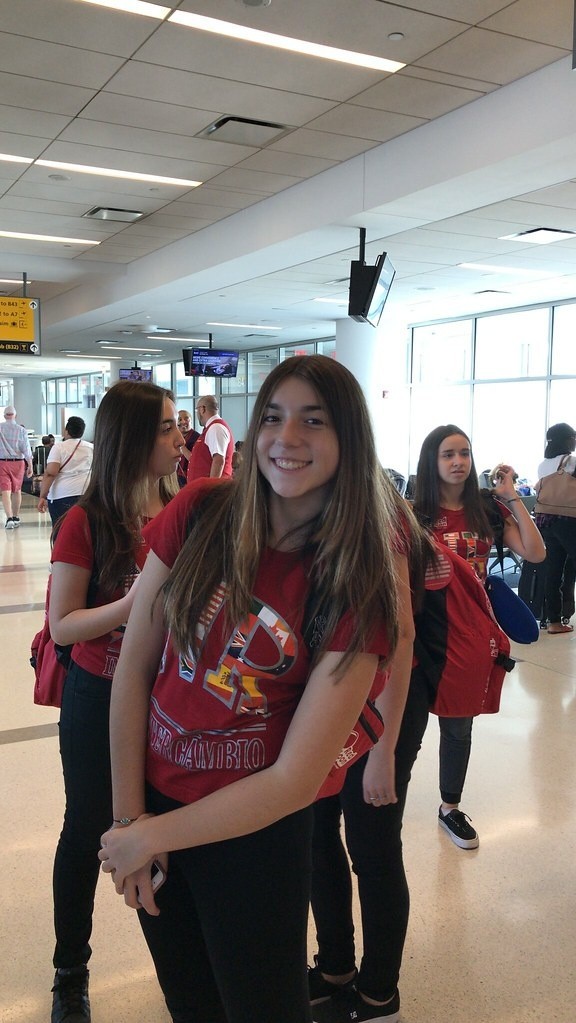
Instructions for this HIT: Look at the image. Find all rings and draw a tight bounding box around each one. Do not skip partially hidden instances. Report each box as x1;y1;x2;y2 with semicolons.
369;797;376;800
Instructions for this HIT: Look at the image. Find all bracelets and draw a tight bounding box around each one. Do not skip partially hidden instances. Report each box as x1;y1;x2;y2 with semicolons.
112;817;137;825
506;496;520;503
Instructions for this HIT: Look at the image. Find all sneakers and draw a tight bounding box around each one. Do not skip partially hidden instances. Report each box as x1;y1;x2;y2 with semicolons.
311;973;400;1023
306;964;358;1006
438;805;480;849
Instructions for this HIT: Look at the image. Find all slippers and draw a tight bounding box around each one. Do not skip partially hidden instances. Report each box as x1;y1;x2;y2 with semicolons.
548;625;573;634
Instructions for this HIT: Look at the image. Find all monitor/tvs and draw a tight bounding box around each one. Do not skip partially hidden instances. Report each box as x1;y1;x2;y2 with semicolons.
119;369;152;382
182;347;239;379
348;252;396;328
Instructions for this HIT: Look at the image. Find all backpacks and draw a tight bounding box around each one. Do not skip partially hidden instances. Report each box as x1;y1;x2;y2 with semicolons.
30;499;101;707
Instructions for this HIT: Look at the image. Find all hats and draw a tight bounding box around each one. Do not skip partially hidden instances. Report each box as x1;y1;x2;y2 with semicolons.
547;423;576;444
4;406;16;416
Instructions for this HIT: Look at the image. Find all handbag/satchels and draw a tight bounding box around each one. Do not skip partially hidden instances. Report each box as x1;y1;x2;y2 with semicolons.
534;454;576;518
482;493;539;644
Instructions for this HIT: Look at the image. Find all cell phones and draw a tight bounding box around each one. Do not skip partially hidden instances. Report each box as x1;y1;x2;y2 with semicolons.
136;861;166;906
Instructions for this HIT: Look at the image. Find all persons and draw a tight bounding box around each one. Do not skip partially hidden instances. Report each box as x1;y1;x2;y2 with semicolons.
491;461;537;498
90;349;392;1023
32;434;55;474
515;424;576;634
37;415;95;545
303;464;447;1023
175;408;201;489
185;393;234;484
384;425;547;850
0;405;34;529
52;372;189;1023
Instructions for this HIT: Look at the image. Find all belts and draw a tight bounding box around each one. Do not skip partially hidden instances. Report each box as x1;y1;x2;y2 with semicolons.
0;459;24;461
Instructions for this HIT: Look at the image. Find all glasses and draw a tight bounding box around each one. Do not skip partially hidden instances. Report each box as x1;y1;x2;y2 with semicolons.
194;405;206;412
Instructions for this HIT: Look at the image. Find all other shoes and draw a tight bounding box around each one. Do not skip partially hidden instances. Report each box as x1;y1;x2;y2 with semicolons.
5;518;14;529
52;964;91;1023
12;517;20;527
